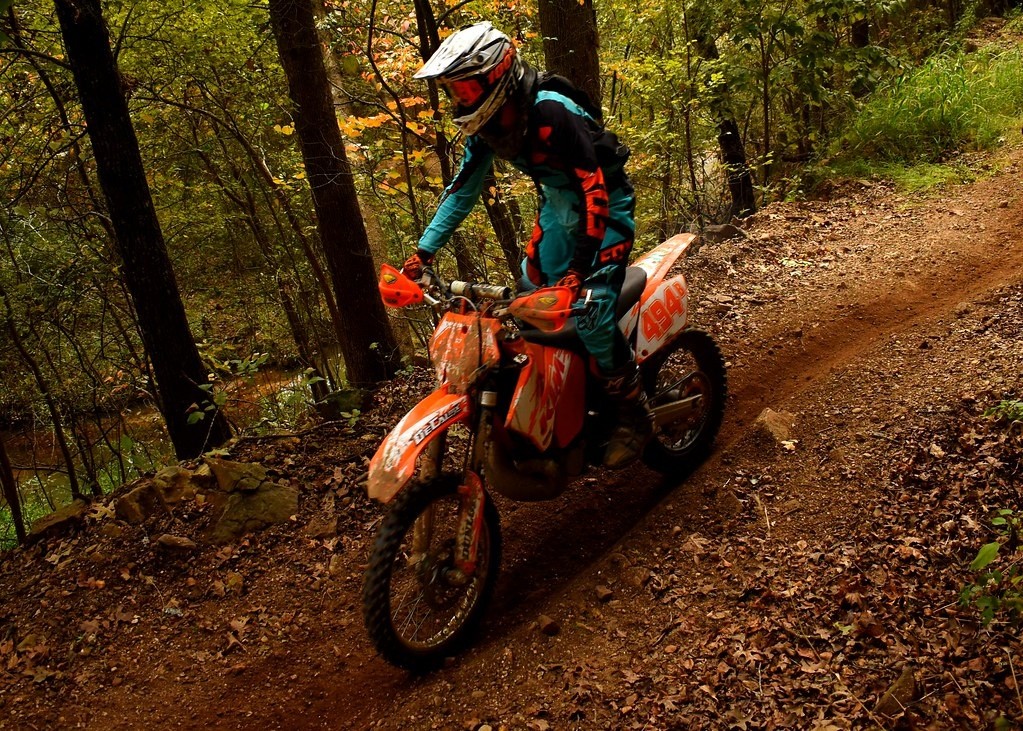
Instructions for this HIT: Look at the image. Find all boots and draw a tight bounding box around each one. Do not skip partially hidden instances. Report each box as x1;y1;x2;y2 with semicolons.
600;350;657;469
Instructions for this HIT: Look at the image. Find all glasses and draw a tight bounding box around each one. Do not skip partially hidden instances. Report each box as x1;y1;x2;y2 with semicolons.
439;74;489;119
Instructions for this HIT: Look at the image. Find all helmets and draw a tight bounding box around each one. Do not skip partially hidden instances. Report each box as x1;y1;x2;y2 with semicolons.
414;20;525;136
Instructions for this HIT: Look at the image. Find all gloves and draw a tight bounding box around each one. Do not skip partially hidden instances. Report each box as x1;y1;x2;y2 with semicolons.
552;270;586;303
405;249;434;280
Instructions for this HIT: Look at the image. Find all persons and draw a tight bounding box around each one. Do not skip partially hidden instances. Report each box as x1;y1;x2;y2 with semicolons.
403;21;655;470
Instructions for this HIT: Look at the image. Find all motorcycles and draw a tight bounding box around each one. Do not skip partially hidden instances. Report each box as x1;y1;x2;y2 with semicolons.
361;233;727;670
